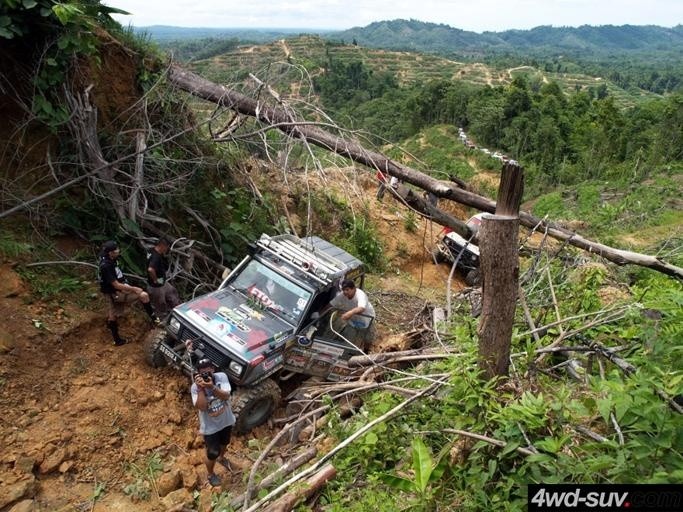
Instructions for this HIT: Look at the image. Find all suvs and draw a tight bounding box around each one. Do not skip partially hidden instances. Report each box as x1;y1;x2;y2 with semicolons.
142;232;370;440
432;211;496;287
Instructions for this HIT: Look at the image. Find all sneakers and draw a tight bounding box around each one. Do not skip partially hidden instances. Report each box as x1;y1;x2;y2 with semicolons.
154;317;167;328
206;472;222;487
216;457;233;468
112;338;131;346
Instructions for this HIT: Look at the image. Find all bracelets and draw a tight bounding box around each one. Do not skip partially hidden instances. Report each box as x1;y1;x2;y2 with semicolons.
211;386;219;393
195;387;204;392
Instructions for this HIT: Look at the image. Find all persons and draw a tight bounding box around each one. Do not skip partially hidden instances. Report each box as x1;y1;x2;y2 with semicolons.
320;279;378;357
145;239;181;318
189;356;237;489
96;241;164;346
376;171;387;202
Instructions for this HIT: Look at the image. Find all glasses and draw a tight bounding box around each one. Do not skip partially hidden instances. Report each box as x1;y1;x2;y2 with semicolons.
198;359;211;365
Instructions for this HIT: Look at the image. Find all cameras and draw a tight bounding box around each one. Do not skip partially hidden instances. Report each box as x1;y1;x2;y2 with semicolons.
201;372;212;382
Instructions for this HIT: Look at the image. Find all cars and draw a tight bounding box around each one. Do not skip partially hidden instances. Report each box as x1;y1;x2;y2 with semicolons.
456;126;520;168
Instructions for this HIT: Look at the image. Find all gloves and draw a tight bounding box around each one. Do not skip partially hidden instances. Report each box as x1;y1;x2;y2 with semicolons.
156;278;164;285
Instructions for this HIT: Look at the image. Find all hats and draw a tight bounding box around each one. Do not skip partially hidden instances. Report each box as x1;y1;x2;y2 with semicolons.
98;239;119;256
